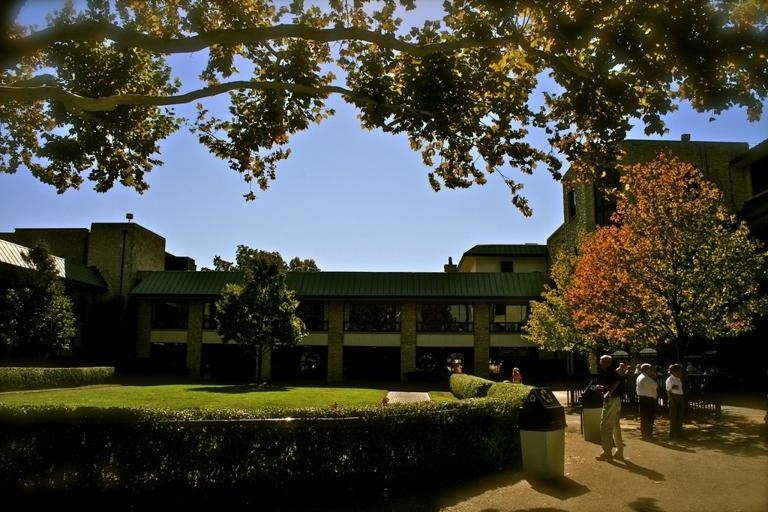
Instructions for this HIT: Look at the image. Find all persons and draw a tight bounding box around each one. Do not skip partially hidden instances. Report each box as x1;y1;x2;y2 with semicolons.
595;355;625;461
615;361;642;376
636;363;659;442
512;367;522;384
666;363;685;441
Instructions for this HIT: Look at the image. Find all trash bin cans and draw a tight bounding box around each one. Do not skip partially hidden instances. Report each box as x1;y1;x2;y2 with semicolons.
516;387;565;480
579;379;604;442
